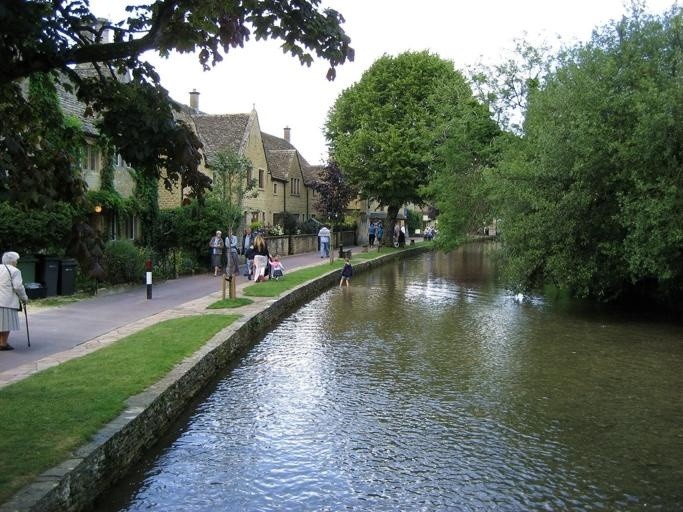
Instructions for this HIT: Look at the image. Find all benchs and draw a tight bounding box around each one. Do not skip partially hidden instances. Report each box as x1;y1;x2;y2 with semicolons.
342;248;352;264
424;237;433;242
400;241;405;248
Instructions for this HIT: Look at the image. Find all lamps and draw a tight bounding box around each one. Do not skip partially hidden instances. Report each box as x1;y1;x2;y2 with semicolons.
94;201;105;216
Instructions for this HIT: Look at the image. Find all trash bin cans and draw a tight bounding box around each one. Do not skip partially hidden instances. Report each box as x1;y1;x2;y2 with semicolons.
17;257;76;297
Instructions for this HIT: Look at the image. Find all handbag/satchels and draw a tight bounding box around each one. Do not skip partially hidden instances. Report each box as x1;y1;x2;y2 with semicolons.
237;249;240;254
17;303;22;311
209;247;213;254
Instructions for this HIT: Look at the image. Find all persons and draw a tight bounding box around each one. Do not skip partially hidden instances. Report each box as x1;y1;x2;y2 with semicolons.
367;223;377;248
0;251;29;350
208;226;285;283
376;222;383;244
398;230;406;247
424;226;435;242
317;224;331;258
339;258;354;287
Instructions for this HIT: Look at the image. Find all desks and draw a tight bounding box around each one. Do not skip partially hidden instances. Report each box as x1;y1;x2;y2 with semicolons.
407;238;417;247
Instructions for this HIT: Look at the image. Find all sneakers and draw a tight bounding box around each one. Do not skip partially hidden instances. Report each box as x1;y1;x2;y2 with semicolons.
0;344;14;350
